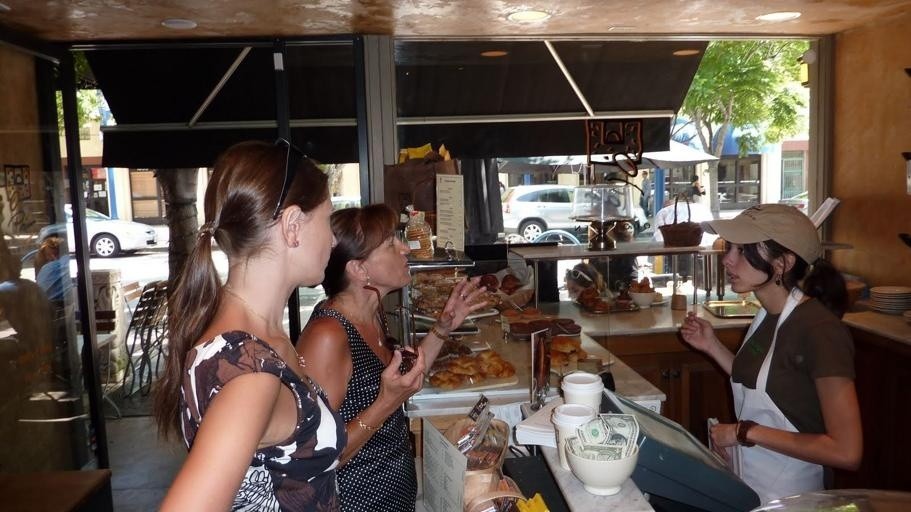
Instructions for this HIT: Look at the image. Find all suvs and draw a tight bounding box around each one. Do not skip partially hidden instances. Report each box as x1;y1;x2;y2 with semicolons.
501;183;651;241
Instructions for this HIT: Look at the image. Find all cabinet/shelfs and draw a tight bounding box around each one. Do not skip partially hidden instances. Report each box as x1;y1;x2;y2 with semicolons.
0;465;115;511
822;327;911;497
593;326;750;448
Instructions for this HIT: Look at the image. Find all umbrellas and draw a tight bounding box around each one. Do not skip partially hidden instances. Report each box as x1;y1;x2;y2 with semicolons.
495;139;722;241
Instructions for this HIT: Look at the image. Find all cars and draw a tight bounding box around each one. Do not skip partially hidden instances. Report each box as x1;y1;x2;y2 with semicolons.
65;203;157;258
777;190;808;218
37;221;66;247
330;196;362;212
651;182;670;201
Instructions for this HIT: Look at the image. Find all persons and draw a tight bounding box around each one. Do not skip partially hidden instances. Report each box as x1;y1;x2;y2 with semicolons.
680;204;863;502
564;263;608;301
297;203;490;511
158;139;347;511
0;235;75;472
640;171;652;220
683;174;707;203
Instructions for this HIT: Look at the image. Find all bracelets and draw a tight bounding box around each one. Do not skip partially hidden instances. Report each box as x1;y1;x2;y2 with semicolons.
738;419;759;447
356;415;384;432
431;326;449;341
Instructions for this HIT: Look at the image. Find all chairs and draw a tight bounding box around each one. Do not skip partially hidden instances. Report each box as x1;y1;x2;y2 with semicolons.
113;277;174;400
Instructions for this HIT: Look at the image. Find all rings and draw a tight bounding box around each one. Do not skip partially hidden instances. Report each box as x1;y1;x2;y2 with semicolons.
460;293;467;300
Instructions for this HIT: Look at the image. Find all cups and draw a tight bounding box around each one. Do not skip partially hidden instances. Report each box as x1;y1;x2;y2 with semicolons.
672;294;688;311
560;373;606;414
552;404;597;471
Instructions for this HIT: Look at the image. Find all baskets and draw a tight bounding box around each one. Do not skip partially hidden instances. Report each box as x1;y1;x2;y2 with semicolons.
657;193;704;249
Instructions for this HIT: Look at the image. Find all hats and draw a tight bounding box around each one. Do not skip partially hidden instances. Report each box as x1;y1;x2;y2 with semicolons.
700;203;825;266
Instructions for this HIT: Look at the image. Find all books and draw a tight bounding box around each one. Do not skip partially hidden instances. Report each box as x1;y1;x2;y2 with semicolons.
708;417;744;480
515;397;566;448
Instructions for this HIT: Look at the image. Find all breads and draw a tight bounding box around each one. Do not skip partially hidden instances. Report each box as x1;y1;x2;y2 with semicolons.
406;271;662;394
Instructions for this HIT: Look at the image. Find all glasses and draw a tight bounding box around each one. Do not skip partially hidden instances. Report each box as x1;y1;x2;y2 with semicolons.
363;285;419;361
272;134;306;224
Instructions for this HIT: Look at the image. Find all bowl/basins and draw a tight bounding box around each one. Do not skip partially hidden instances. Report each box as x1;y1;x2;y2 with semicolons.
564;441;640;496
629;290;658;310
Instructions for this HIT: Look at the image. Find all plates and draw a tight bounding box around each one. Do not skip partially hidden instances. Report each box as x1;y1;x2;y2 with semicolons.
869;285;911;314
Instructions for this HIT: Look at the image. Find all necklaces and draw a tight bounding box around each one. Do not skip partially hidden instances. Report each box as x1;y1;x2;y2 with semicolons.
334;292;383;347
221;286;307;369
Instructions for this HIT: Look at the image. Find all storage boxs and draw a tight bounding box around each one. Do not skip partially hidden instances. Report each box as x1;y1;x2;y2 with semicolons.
380;156;467;231
